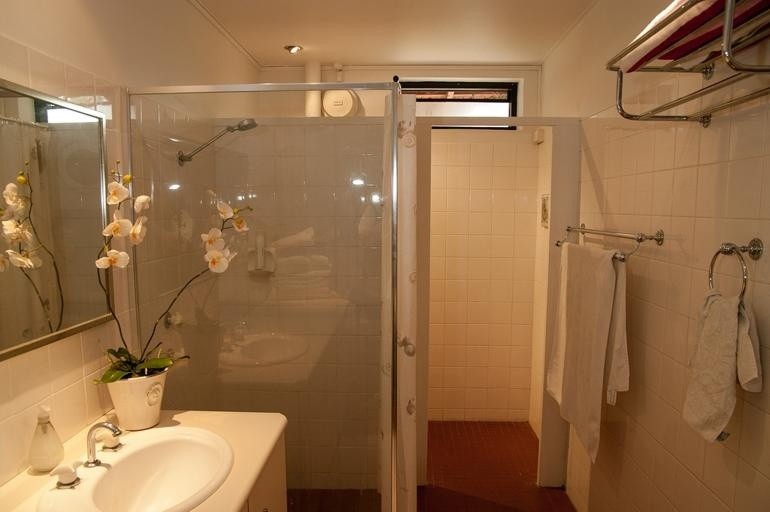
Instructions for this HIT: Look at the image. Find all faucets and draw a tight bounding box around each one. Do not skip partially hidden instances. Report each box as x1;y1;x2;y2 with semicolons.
226;317;248;352
85;420;122;470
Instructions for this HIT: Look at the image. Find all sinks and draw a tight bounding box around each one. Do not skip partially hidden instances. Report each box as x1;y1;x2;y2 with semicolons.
83;424;233;512
217;330;311;368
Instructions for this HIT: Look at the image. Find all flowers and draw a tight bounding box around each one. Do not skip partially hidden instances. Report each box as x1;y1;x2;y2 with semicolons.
0;162;63;336
90;170;254;386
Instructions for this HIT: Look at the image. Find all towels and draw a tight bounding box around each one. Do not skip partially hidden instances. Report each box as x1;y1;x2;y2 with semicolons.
545;240;632;464
680;290;764;443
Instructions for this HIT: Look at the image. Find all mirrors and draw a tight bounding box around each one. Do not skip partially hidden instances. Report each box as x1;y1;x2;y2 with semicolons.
0;76;117;359
